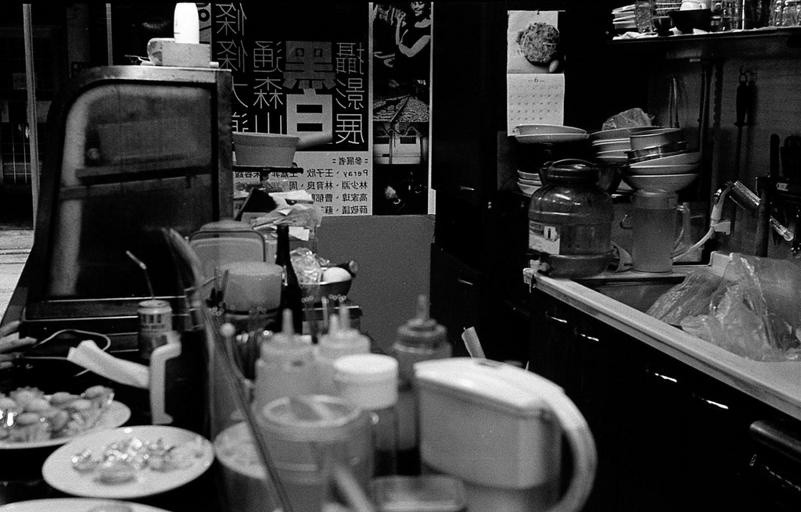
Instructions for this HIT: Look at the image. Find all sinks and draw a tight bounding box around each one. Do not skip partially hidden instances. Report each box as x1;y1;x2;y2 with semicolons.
574;275;801;364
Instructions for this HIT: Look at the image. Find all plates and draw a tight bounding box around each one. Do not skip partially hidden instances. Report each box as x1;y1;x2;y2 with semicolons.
513;168;542;199
591;123;705;176
0;497;167;511
518;22;559;68
213;424;263;483
511;122;590;144
40;426;212;501
0;393;132;454
609;5;635;32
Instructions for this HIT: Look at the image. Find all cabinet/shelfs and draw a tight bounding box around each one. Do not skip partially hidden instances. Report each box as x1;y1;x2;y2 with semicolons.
530;285;749;511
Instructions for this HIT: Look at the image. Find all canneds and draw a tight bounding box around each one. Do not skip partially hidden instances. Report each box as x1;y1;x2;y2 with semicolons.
138;300;172;360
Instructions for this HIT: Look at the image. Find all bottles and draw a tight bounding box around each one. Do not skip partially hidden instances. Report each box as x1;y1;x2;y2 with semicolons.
330;352;401;469
251;308;316;413
317;306;371;385
388;291;454;456
767;0;801;26
249;391;374;511
271;223;306;325
321;261;361;281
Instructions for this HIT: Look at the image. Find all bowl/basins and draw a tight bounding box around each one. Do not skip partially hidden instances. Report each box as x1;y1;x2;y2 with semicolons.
229;131;301;169
621;175;700;195
299;277;353;310
665;7;716;34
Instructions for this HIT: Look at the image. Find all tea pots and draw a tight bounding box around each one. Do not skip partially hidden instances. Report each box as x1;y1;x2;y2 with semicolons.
628;187;690;274
415;357;599;511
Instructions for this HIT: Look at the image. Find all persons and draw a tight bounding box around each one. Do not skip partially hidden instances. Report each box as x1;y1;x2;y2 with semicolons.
393;1;432;72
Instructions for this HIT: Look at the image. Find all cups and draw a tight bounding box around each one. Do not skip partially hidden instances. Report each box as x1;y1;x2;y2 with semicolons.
172;2;200;46
651;16;674;36
